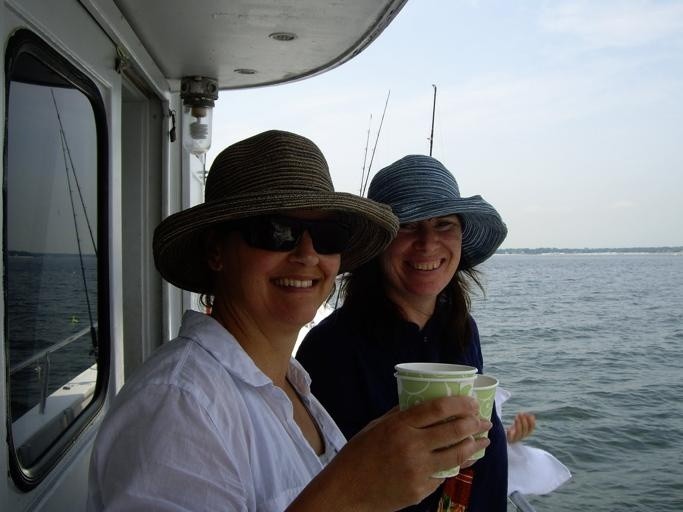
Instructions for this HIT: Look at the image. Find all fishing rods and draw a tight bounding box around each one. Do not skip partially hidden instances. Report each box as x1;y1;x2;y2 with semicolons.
50;88;97;255
60;129;98;356
334;90;391;309
427;85;436;156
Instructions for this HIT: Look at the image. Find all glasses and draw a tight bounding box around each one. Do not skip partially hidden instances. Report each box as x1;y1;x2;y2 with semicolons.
244;212;347;255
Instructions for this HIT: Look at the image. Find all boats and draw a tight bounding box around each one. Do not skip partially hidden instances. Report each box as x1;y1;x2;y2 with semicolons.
0;1;409;512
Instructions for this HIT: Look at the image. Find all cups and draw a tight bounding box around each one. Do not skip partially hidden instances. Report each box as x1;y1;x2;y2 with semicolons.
466;374;499;459
394;361;478;377
395;373;476;477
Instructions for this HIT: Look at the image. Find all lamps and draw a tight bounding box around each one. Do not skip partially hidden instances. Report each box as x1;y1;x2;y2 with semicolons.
176;74;218;156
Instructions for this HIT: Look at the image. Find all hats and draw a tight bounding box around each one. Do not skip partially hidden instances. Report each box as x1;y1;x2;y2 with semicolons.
368;154;508;270
152;130;398;296
